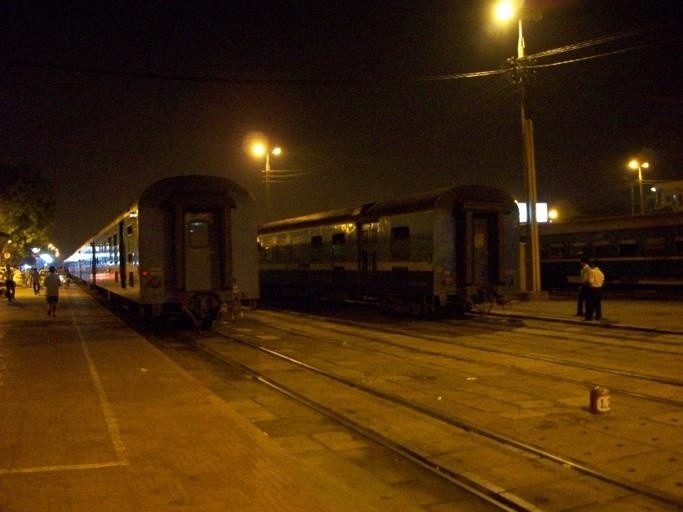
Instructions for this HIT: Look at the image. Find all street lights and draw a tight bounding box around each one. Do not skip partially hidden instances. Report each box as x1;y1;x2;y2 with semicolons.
487;1;543;292
629;160;650;213
244;133;282;222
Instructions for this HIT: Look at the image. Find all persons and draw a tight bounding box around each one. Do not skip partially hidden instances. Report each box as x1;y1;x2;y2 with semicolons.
583;260;605;321
29;268;41;294
573;257;590;317
26;266;34;288
1;263;15;302
43;264;61;317
62;266;70;287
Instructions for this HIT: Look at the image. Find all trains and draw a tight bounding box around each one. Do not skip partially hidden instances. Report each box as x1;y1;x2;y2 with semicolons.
257;185;544;322
64;175;256;332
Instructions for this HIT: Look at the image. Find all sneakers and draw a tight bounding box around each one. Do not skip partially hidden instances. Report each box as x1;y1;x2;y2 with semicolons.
34;290;40;295
47;309;51;316
52;314;56;317
6;296;15;304
575;312;601;321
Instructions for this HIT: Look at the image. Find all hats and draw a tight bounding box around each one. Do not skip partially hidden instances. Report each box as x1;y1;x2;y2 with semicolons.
49;266;56;271
